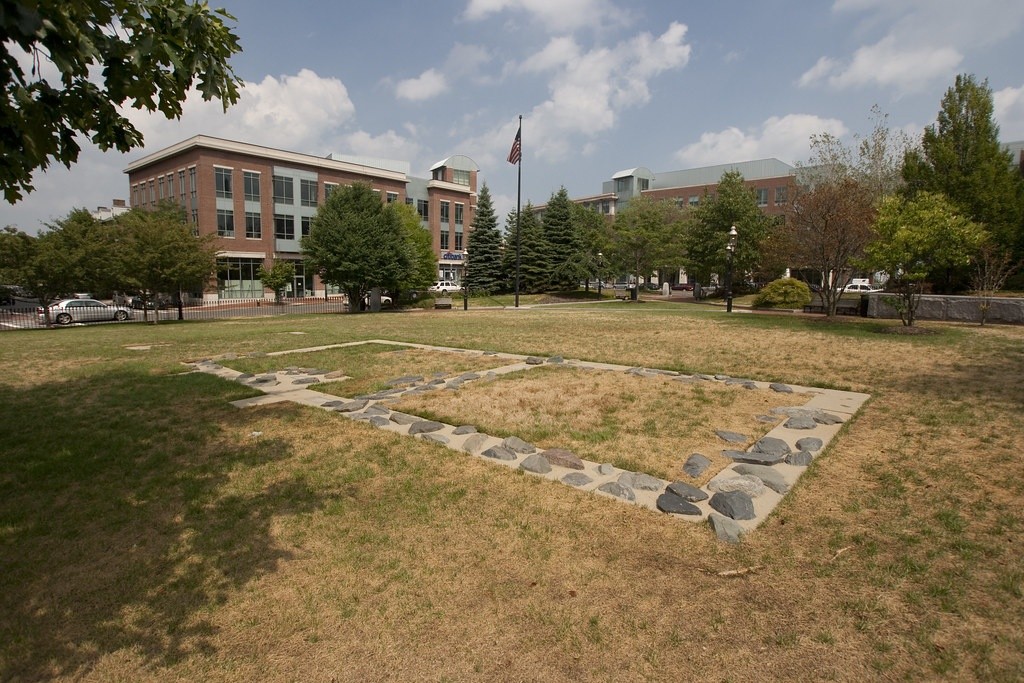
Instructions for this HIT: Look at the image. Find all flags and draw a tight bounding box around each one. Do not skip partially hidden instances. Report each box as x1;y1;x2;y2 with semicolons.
506;128;521;164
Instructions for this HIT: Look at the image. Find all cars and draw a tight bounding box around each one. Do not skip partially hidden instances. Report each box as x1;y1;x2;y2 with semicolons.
671;283;693;291
428;279;465;296
612;281;636;290
132;293;166;310
639;283;659;293
37;298;134;325
113;290;143;307
838;283;883;294
580;281;613;289
342;291;393;306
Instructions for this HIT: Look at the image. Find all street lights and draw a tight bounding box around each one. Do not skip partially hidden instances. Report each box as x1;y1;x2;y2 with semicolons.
727;226;738;312
597;252;603;301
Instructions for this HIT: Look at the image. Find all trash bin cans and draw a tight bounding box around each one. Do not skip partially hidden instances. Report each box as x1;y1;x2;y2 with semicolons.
693;282;701;296
860;294;869;316
631;288;637;299
